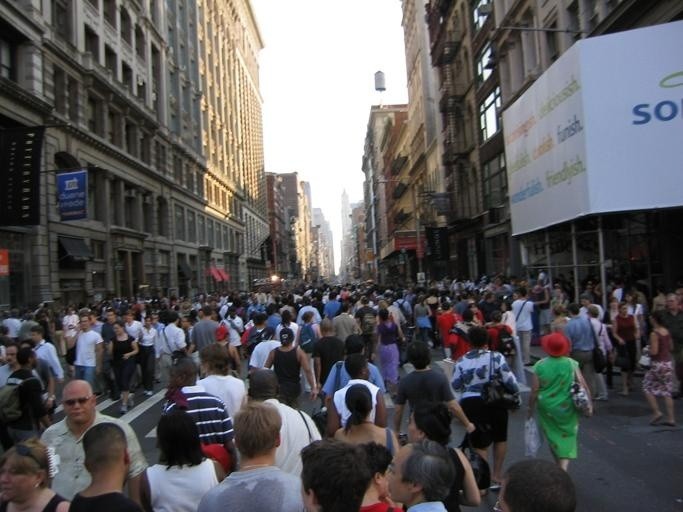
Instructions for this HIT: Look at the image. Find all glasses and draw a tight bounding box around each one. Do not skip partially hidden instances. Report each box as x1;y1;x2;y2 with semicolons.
63;395;92;405
493;500;504;512
16;443;44;468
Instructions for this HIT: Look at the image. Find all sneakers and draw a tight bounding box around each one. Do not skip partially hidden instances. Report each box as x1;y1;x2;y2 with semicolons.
593;396;609;401
120;404;129;413
146;390;153;396
128;395;135;408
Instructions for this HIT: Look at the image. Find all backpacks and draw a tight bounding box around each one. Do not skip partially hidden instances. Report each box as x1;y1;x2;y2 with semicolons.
494;325;518;357
395;300;406;316
0;376;39;421
362;311;376;335
299;322;318;353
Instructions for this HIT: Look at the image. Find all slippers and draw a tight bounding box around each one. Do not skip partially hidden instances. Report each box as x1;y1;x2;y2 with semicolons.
489;484;503;490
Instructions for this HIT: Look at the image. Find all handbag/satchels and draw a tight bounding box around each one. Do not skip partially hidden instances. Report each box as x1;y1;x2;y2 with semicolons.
481;351;520;411
311;360;343;437
588;317;606;373
162;327;185;365
200;442;233;474
457;432;491;490
614;344;631;370
638;353;653;370
64;338;78;366
568;358;593;417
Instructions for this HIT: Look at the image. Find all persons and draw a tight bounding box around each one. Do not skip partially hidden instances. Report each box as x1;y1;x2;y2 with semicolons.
532;285;552;335
88;311;104;335
436;302;460;359
387;438;457;512
68;421;143;512
138;315;160;397
296;298;322;324
247;368;322;478
479;292;498;322
586;305;613;401
355;296;378;364
550;305;572;335
452;294;470;315
266;303;283;330
322;333;387;409
449;326;523;497
218;291;229;307
20;339;56;429
73;315;104;394
438;289;451;308
232;298;246;318
0;325;13;365
486;310;513;351
139;409;220;512
387;297;408;325
62;307;79;349
356;441;405;512
108;320;140;414
652;285;665;311
468;303;482;326
124;310;144;394
219;306;244;360
377;289;393;313
180;315;200;370
392;340;477;438
263;327;319;404
158;311;187;389
609;280;623;322
429;274;491;295
189;306;219;353
80;292;219;313
579;284;595;304
248;326;283;373
196;343;248;425
511;286;534;367
537;269;548;285
494;458;577;512
225;289;249;323
658;293;683;399
196;400;306;512
371;308;404;385
218;295;233;319
17;313;40;342
526;288;540;337
213;326;241;378
491;273;508;299
275;309;299;346
3;308;21;343
602;296;620;389
408;400;481;512
499;300;528;385
327;354;387;435
160;357;238;476
613;302;639;395
312;318;345;408
30;326;64;414
37;313;51;342
246;296;267;322
448;309;489;360
143;312;165;383
624;290;646;371
6;347;52;445
392;291;413;363
294;310;322;394
376;299;396;326
0;436;71;512
640;310;679;427
39;379;149;512
425;288;441;349
563;303;599;400
241;313;268;377
101;309;125;401
278;297;297;322
527;332;592;473
300;438;376;512
333;383;400;457
0;343;44;452
322;292;342;320
578;292;604;322
549;286;570;318
256;285;279;306
333;303;358;344
280;281;378;300
413;295;434;350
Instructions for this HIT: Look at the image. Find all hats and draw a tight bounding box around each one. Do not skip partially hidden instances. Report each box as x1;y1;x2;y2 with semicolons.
531;285;544;294
215;325;229;340
541;332;571;357
280;328;294;342
